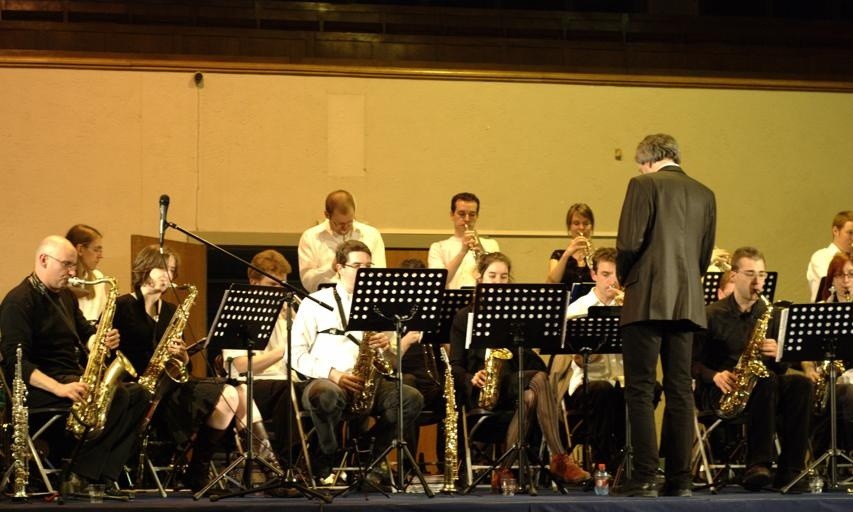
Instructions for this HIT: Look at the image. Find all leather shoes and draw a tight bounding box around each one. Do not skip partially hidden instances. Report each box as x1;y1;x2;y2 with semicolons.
105;486;135;498
66;476;103;497
779;470;806;486
661;481;692;496
610;479;659;499
743;463;772;492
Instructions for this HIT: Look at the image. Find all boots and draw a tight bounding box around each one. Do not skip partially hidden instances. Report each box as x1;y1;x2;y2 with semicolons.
239;421;302;496
183;421;235;496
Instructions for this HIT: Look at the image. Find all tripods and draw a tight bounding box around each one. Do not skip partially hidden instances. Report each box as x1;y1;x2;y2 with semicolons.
418;289;476;345
168;222;333;504
542;313;626;492
193;284;313;500
782;302;852;496
333;269;448;500
459;283;570;496
609;400;666;496
701;272;777;484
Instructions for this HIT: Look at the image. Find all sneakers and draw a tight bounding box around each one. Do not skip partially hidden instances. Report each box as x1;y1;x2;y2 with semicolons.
499;467;515;490
549;453;590;483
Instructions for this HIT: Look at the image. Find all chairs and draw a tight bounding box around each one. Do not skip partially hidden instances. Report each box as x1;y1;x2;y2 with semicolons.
0;362;74;503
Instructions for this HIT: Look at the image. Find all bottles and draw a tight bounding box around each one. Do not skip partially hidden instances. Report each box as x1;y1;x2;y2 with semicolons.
594;464;609;496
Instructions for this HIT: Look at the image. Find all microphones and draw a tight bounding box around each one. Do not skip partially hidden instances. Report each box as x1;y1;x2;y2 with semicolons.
158;194;169;246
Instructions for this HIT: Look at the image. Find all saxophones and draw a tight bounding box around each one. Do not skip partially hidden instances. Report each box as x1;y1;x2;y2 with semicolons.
813;362;852;412
478;348;513;410
351;331;394;417
140;281;199;400
719;290;774;418
65;276;138;442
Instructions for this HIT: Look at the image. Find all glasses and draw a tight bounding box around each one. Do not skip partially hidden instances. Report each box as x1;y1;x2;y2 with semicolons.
832;272;853;280
339;262;375;270
736;269;768;280
331;215;356;228
45;252;78;272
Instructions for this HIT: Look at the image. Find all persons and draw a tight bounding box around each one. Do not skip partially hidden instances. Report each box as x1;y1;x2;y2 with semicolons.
694;249;813;488
615;133;717;498
566;247;662;455
701;247;730;283
297;190;387;295
285;239;424;482
0;235;130;484
393;258;464;475
112;244;283;496
64;223;106;349
806;210;853;304
222;249;308;415
800;252;852;472
427;191;498;289
548;201;595;301
449;252;590;492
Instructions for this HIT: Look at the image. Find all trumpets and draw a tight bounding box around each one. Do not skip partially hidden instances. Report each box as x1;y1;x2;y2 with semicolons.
579;231;602;270
463;224;493;265
